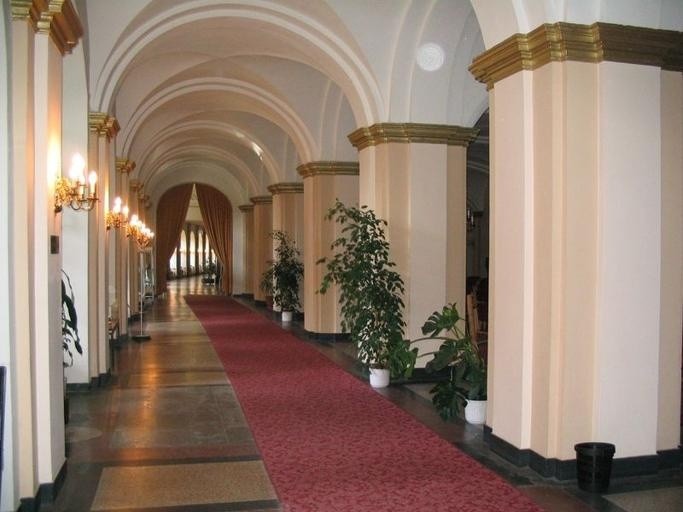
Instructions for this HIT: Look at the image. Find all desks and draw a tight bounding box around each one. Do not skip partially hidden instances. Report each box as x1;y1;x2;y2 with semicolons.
108;318;119;342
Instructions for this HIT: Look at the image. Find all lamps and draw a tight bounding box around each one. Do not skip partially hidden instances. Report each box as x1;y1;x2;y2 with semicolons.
106;198;155;249
52;152;98;213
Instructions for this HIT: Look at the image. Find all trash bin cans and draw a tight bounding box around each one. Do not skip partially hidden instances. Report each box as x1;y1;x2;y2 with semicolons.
575;442;615;493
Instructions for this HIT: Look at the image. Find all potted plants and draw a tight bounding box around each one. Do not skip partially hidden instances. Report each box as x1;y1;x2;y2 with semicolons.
390;302;487;425
260;230;304;323
315;198;409;387
